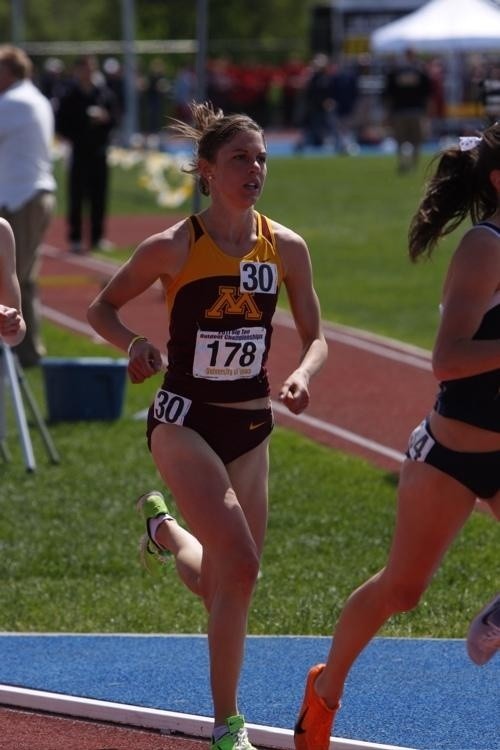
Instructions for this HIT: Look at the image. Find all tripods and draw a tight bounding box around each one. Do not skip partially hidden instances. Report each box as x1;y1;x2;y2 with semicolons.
0;341;60;471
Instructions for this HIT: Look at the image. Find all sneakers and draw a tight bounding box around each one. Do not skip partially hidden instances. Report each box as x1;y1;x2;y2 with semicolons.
135;490;173;579
211;714;259;750
466;595;499;667
294;664;342;749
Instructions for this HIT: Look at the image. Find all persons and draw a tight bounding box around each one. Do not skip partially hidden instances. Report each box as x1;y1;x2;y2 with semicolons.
86;99;327;750
66;47;383;160
1;42;60;370
377;46;500;174
1;217;27;348
53;48;126;258
292;120;500;750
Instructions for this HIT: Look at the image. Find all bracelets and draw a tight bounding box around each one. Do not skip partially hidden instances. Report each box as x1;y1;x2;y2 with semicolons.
126;335;148;358
